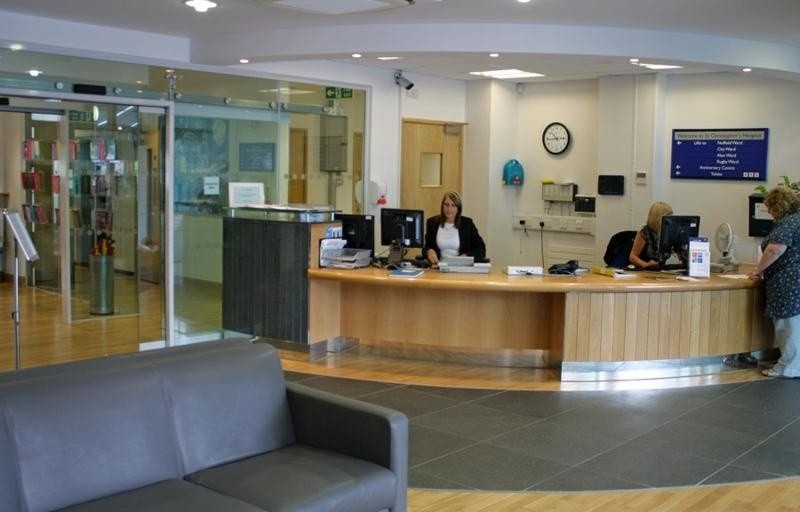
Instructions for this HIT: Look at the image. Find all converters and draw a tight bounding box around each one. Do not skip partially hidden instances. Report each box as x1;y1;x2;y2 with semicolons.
519;220;524;224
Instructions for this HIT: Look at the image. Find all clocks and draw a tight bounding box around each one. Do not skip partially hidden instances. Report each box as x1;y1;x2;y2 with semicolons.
542;122;570;155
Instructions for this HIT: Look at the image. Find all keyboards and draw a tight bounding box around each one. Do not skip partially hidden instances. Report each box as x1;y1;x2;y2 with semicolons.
645;263;686;271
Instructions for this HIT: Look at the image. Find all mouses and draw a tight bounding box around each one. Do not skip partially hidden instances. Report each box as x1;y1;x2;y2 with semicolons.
627;263;636;270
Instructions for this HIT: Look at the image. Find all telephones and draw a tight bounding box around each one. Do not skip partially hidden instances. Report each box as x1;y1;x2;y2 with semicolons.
548;259;578;274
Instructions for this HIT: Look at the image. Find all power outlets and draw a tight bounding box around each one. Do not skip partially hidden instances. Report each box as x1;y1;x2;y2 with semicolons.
519;218;584;230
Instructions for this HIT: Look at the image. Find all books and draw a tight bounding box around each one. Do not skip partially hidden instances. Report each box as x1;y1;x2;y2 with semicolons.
389;269;424;277
592;265;637;278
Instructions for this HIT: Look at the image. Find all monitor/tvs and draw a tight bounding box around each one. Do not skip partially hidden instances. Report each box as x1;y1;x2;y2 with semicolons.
659;214;700;272
380;208;425;268
334;214;374;259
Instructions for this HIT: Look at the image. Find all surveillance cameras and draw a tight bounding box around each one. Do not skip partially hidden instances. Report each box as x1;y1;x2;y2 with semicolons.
396;76;415;91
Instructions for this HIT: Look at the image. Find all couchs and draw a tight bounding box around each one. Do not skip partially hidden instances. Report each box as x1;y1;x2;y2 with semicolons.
0;337;408;512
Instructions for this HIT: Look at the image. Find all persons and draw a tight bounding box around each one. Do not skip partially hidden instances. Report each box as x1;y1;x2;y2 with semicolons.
422;190;486;267
747;185;800;381
629;202;674;267
720;352;759;368
